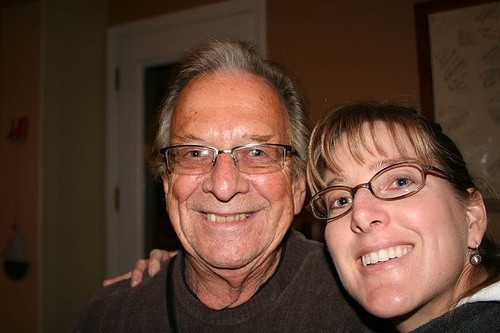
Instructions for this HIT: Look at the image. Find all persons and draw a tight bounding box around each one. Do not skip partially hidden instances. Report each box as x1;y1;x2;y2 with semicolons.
71;36;403;333
102;100;500;333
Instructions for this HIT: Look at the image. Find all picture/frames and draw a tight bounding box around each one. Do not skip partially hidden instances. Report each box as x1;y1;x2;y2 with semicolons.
414;0;500;212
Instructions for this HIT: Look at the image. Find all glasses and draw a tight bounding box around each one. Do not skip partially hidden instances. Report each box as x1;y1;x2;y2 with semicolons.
160;144;294;176
306;161;453;221
